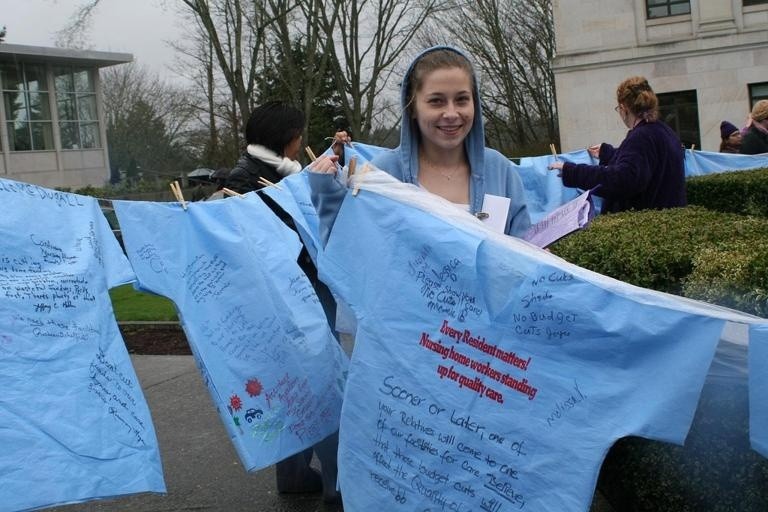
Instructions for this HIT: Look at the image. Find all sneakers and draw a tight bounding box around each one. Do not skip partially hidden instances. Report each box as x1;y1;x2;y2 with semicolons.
276;467;343;511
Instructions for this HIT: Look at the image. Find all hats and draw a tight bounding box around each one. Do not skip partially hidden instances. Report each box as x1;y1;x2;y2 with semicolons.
720;120;739;140
751;99;768;121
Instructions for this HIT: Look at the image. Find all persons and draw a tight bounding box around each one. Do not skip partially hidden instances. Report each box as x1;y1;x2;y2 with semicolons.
740;99;768;154
546;75;688;216
676;130;696;150
305;43;533;249
223;100;351;511
720;121;742;154
739;112;754;137
